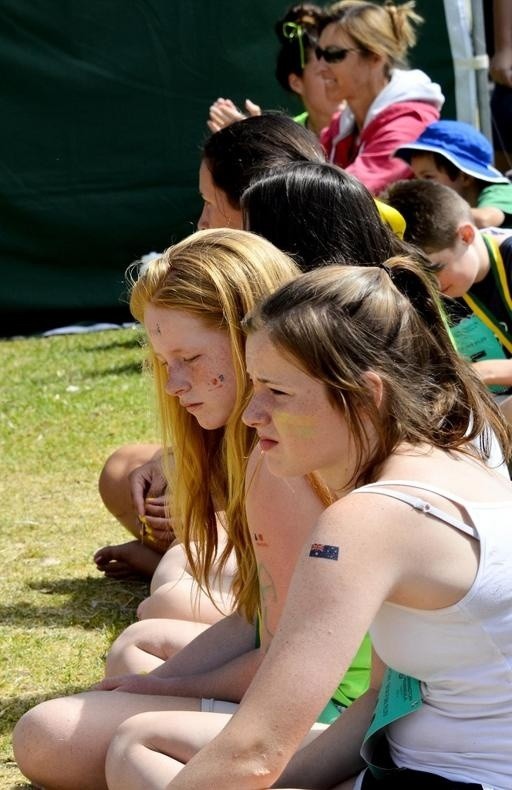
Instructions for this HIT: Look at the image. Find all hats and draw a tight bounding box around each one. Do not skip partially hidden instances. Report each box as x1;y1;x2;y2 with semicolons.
390;118;511;186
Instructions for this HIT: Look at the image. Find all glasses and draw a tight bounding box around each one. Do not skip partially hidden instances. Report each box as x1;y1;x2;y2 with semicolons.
314;45;363;63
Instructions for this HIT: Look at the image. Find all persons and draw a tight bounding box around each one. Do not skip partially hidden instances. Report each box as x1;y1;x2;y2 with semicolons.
11;0;512;789
